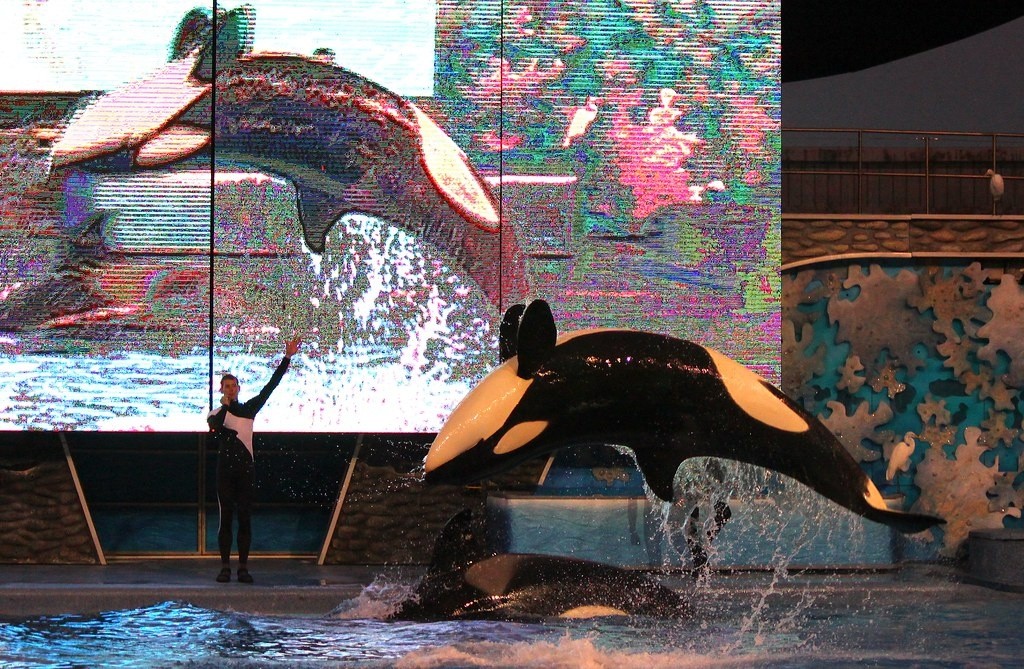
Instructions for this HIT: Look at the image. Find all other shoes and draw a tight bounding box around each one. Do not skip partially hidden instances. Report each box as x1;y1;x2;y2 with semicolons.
216;567;232;582
236;568;254;584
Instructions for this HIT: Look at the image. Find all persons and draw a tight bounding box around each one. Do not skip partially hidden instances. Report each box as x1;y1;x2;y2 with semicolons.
207;336;303;583
